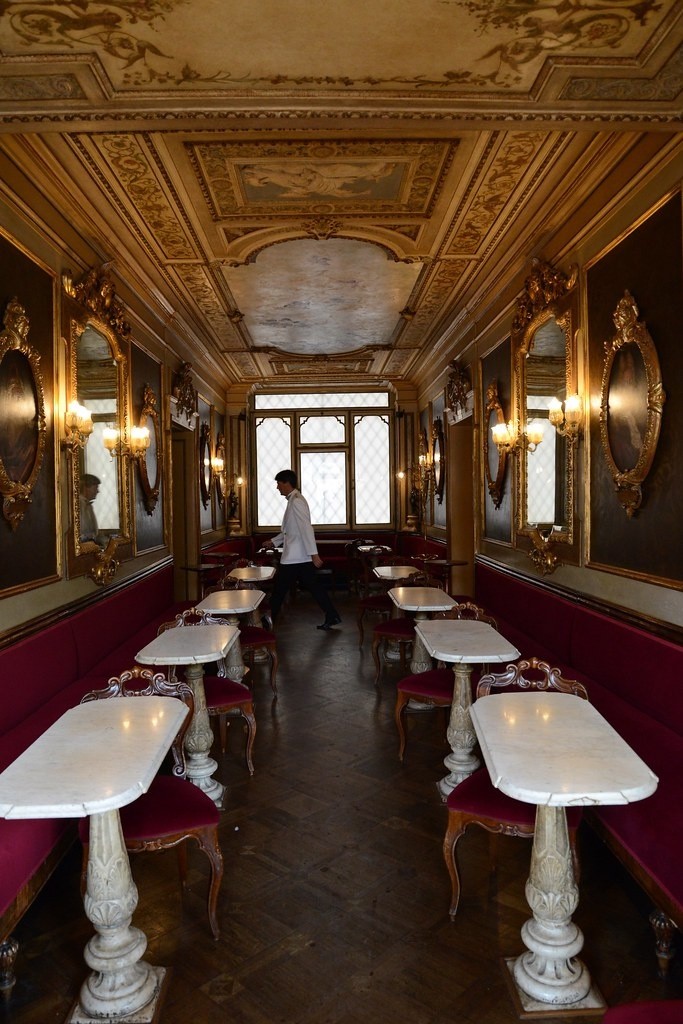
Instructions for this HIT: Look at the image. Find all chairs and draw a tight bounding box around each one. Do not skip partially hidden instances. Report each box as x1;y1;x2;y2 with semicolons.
343;537;590;917
78;547;279;941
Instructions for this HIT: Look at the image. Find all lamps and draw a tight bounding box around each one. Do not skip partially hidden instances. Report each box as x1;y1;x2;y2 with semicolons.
228;474;243;520
130;424;150;461
61;400;94;459
102;428;118;462
491;423;516;457
547;394;583;444
212;457;223;472
396;468;419;515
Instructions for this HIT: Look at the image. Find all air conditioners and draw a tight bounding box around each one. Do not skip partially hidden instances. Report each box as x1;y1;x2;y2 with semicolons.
526;424;543;455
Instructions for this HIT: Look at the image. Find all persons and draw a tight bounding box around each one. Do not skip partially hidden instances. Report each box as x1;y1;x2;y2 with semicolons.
262;470;342;629
79;474;101;537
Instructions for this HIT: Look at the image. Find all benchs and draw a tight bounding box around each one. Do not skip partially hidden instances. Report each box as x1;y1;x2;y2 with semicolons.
448;553;683;983
200;529;449;591
0;553;200;1011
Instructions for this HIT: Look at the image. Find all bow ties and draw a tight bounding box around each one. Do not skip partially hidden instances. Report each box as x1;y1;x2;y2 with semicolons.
86;501;93;505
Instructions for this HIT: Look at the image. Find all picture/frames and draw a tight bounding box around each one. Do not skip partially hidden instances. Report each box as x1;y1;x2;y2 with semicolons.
0;295;48;534
599;289;666;519
217;432;227;509
425;416;444;506
482;377;505;511
138;381;164;516
200;421;214;506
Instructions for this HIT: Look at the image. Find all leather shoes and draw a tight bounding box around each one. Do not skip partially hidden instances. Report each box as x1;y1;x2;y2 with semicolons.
317;615;342;629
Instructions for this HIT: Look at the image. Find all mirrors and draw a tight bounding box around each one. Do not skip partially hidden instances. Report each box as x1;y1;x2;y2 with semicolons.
70;312;133;590
512;306;575;578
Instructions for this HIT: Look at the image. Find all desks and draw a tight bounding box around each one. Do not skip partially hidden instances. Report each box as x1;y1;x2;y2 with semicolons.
412;620;522;804
196;591;267;718
134;625;243;812
0;696;190;1024
371;566;425;663
387;587;460;714
424;560;468;592
180;563;225;599
203;552;240;577
227;567;277;662
358;545;392;614
468;692;660;1020
